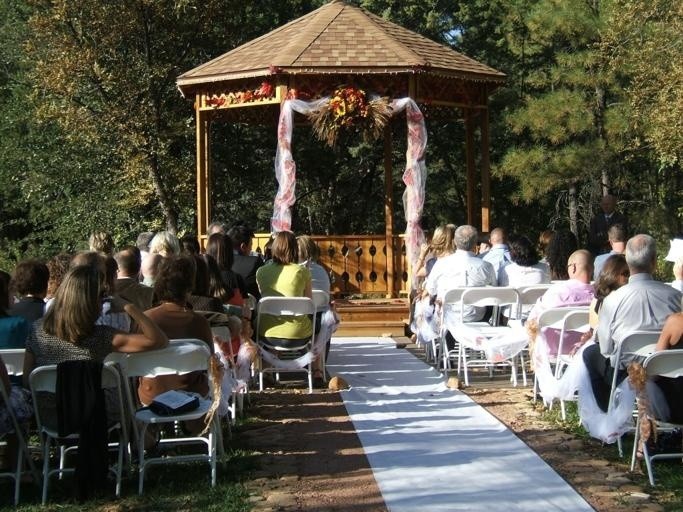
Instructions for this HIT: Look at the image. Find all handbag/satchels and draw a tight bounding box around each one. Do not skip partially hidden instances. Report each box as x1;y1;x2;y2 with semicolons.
149;389;200;417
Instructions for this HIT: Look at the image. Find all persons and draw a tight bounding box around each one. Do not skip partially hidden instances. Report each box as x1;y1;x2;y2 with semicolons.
1;221;339;465
410;220;683;459
590;193;629;233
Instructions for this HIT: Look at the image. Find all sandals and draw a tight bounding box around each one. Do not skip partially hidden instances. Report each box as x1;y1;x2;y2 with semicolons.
312;369;323;388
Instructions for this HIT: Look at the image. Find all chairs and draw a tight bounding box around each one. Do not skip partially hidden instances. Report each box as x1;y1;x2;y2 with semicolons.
514;284;683;487
410;285;520;387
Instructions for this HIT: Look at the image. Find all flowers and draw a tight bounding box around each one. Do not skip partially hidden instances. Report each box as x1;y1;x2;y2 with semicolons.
326;85;371;133
